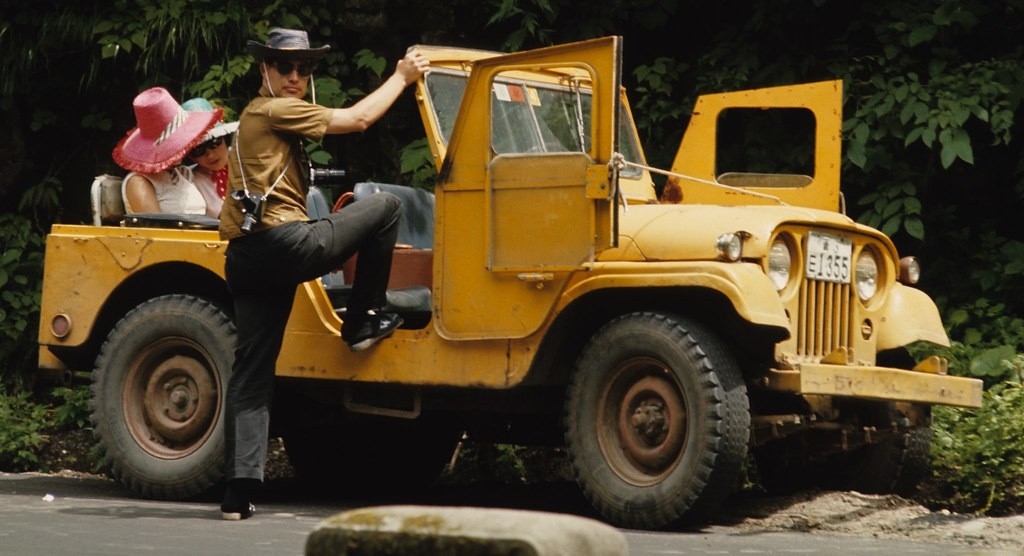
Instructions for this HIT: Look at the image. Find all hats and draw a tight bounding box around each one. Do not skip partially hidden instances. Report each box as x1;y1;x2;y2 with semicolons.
182;98;240;148
246;29;331;66
112;87;223;174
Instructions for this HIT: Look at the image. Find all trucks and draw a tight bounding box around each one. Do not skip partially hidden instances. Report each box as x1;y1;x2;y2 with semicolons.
38;21;986;532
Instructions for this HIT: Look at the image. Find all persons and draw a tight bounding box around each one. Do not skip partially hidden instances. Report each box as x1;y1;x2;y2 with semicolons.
113;87;241;228
218;27;431;520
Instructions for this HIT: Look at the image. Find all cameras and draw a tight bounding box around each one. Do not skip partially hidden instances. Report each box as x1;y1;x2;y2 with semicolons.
230;189;266;234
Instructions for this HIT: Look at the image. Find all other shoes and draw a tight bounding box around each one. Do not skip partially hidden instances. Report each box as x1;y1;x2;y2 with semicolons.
341;311;404;352
221;487;255;520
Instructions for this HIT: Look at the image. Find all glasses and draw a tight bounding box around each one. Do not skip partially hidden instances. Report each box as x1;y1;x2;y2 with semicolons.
272;60;313;76
189;136;222;158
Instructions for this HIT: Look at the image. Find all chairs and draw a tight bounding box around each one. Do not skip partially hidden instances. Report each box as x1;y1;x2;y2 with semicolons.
305;183;435;311
